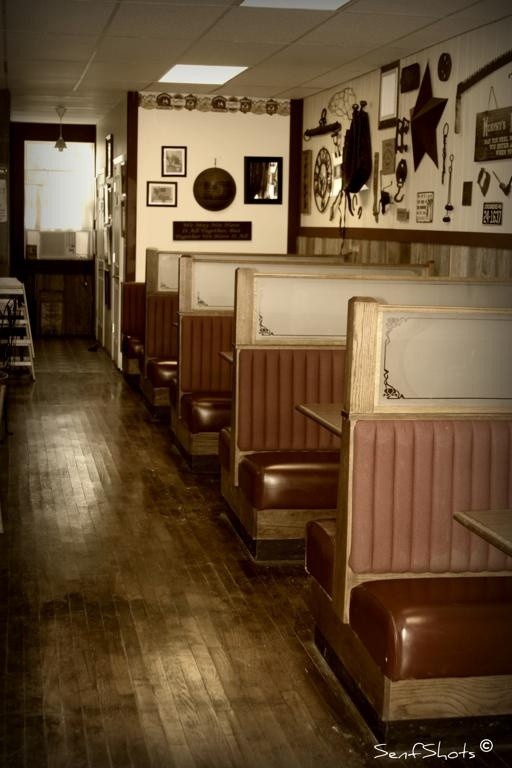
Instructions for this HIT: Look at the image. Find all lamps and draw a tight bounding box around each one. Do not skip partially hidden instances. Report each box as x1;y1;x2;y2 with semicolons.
54;102;68;153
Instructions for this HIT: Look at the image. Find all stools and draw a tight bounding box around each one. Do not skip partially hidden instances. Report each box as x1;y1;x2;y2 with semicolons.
0;276;37;382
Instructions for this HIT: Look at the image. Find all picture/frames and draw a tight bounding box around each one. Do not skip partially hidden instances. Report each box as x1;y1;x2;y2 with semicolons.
162;145;187;177
146;180;178;207
94;134;126;237
244;155;283;205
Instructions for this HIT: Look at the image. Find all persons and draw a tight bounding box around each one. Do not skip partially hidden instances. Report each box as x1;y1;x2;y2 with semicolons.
254;168;277;199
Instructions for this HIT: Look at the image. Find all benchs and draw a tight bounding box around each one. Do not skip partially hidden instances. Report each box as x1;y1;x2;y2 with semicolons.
218;268;512;566
120;246;344;469
307;295;512;748
